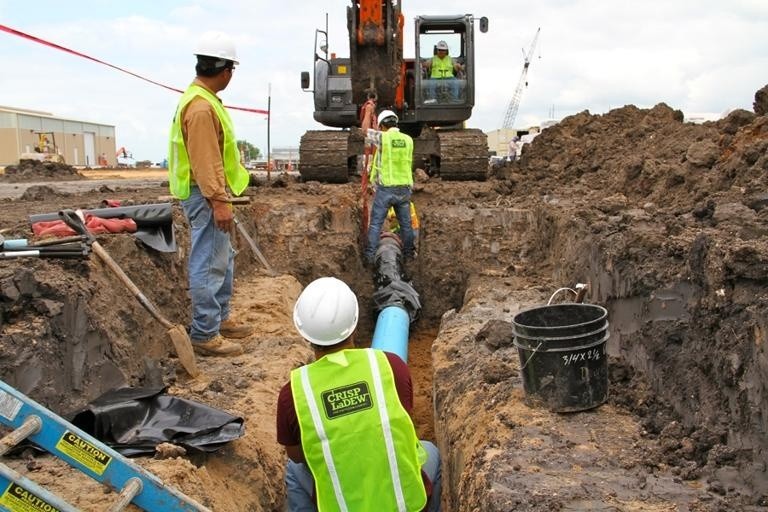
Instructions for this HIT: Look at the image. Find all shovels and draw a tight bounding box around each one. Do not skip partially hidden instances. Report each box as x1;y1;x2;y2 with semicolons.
58;209;199;379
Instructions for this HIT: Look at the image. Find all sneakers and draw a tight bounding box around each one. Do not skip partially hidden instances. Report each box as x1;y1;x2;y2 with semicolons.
424;98;438;104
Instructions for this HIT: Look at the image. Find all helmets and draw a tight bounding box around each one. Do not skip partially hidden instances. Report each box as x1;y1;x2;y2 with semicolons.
293;276;360;346
436;41;449;49
378;110;399;128
191;30;240;66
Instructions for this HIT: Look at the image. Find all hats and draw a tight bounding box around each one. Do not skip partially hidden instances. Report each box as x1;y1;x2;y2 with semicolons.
192;321;254;358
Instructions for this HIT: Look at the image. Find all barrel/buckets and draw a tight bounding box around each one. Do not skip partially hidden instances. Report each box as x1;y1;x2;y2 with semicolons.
510;304;610;414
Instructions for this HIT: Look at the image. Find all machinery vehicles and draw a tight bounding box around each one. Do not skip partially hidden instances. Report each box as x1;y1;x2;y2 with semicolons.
298;0;490;182
501;25;543;130
19;132;65;165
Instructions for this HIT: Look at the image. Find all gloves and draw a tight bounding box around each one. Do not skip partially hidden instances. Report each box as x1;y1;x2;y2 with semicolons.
212;201;234;234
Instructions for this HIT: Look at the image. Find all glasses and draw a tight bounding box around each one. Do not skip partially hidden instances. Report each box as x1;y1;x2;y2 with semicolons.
438;49;445;51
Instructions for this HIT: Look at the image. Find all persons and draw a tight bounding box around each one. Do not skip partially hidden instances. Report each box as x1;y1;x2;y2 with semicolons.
359;99;419;266
169;36;255;358
422;41;464;104
277;277;441;512
509;136;521;163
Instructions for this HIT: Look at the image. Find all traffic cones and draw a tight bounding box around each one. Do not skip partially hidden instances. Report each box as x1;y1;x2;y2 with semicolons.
286;160;294;172
